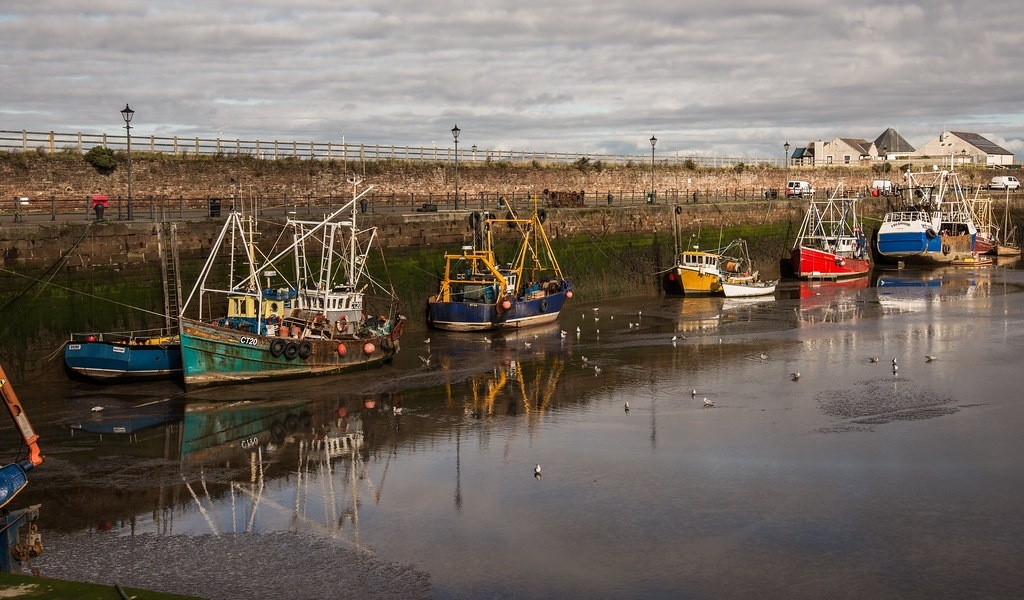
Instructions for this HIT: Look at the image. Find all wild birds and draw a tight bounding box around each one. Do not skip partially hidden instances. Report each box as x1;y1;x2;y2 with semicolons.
625;401;630;408
392;407;402;412
869;357;878;362
419;355;432;363
790;372;800;378
576;308;642;333
692;389;696;394
423;338;431;344
668;335;676;340
580;356;602;371
561;330;568;338
925;355;936;360
525;342;530;346
760;354;767;359
91;406;103;412
534;464;541;473
704;398;714;406
892;358;897;362
892;364;898;369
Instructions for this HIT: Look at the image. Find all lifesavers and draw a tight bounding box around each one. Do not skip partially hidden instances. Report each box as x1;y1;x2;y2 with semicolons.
540;300;549;312
979;219;984;224
469;211;482;229
338;418;350;432
507;211;518;228
943;244;951;254
337;315;350;332
285;416;299;433
268;315;281;327
550;283;557;293
301;416;313;433
537;209;546;224
284;342;299;360
272;423;285;444
677;206;682;214
298;341;312;359
270;338;287;357
926;229;936;239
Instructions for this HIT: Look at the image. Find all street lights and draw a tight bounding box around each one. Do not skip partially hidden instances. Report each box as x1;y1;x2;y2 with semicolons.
451;123;461;209
961;148;966;193
883;146;888;196
120;102;136;219
783;141;790;186
650;135;658;205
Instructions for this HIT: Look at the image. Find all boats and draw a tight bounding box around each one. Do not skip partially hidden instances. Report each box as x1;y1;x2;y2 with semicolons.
790;181;871;279
426;195;573;331
664;291;776;336
662;245;759;296
719;278;780;298
876;155;1021;264
64;325;182;381
177;172;405;383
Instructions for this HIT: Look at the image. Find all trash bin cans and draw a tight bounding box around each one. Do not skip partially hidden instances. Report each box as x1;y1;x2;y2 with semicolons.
210;197;222;217
771;189;777;199
646;193;655;205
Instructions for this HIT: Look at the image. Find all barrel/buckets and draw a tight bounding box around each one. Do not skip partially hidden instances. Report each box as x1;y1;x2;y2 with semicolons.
771;192;777;199
647;194;655;205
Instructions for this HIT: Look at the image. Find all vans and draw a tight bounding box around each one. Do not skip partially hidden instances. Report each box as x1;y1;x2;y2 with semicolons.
992;176;1021;190
786;181;816;198
